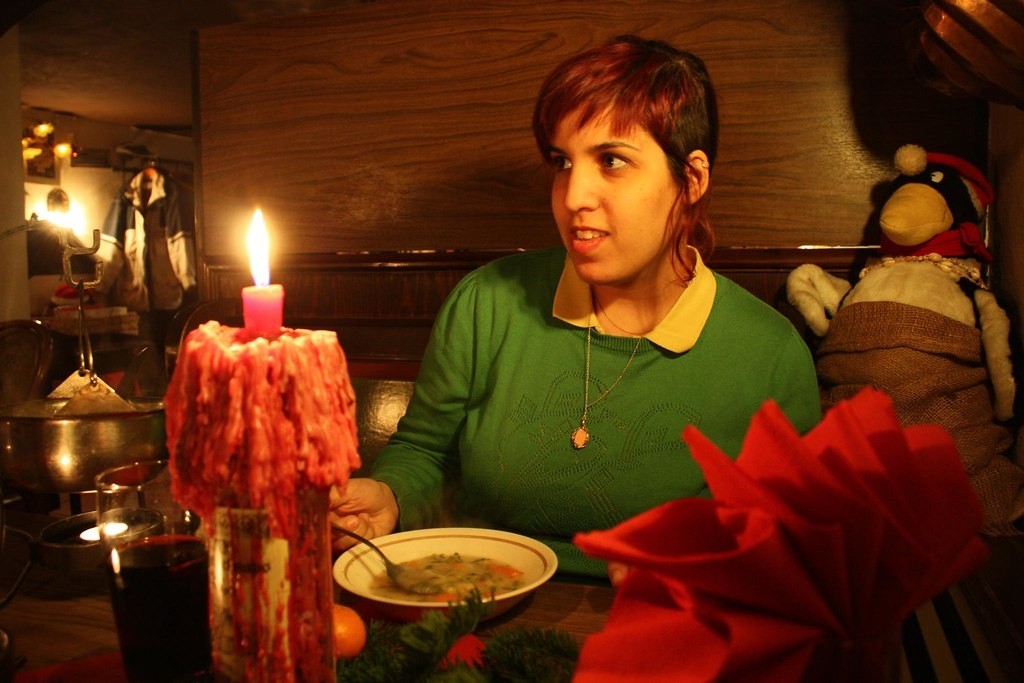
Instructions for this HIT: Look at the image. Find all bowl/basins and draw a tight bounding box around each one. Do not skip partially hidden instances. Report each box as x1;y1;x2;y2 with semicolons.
332;526;559;628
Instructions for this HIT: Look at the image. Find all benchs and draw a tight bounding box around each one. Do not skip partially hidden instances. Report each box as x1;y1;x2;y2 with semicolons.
178;243;886;482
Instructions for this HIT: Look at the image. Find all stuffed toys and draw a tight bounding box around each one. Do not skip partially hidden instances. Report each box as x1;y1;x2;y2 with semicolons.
787;139;1024;539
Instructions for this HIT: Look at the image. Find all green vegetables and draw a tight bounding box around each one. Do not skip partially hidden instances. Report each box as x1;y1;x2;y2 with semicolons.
378;551;521;600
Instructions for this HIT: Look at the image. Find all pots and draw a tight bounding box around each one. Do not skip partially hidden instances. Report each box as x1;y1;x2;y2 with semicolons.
0;278;165;494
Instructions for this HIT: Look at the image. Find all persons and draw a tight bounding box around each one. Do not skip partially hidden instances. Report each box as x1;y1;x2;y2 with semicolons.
328;35;823;590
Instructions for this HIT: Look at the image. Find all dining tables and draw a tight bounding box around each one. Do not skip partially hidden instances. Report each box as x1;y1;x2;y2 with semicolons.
0;571;816;683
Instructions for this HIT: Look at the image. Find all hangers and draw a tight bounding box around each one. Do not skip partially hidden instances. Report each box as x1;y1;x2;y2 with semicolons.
142;154;159;189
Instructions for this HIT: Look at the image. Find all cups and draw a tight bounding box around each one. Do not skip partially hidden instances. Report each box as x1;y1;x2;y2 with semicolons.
94;458;216;683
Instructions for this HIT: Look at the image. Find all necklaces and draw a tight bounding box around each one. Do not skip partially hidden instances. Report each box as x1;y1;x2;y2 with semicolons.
568;293;644;447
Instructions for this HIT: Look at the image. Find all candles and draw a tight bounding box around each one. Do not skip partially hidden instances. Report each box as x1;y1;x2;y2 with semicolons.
239;208;285;338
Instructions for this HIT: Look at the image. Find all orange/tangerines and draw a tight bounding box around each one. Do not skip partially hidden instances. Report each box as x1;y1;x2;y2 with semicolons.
333;604;367;659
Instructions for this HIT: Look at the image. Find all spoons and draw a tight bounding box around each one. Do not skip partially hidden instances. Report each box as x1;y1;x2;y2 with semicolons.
332;523;445;595
